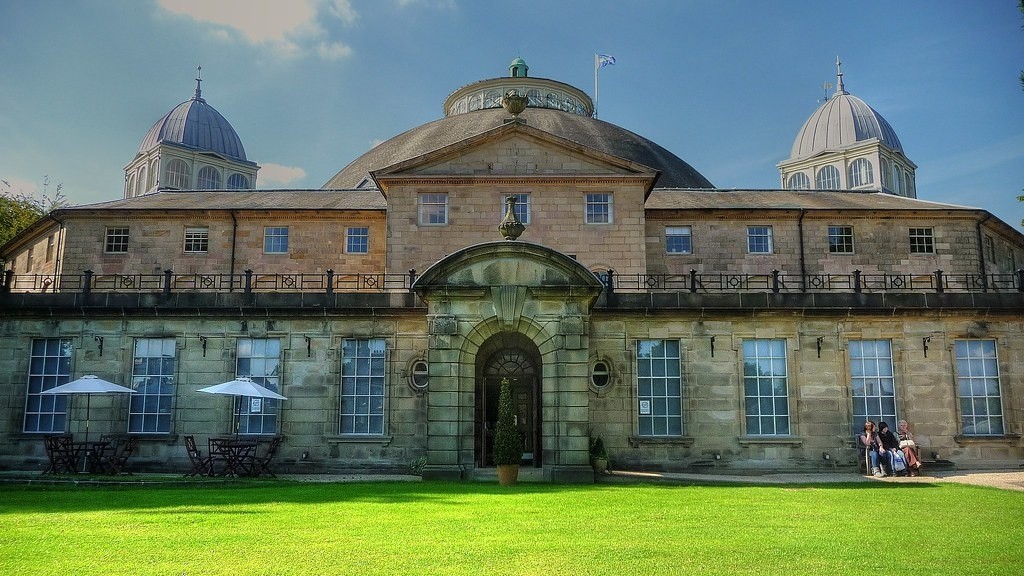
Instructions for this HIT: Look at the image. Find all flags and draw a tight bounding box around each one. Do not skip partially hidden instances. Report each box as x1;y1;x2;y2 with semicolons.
596;54;615;69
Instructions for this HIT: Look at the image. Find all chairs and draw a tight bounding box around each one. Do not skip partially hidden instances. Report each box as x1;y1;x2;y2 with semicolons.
182;432;280;478
42;431;139;476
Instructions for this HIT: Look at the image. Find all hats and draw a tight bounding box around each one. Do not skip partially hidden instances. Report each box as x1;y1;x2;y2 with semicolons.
879;422;888;432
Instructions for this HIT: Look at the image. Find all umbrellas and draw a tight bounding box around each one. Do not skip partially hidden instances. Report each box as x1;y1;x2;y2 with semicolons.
39;375;137;472
195;378;288;474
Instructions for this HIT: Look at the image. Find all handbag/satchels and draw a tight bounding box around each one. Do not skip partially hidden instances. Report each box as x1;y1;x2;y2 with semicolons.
893;451;905;471
899;440;915;449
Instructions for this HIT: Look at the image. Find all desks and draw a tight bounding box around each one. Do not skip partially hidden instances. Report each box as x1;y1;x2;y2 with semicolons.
212;441;261;477
63;442;111;474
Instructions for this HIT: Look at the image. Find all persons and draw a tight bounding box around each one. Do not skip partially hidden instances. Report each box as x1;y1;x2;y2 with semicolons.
878;422;909;476
893;420;922;477
860;421;888;477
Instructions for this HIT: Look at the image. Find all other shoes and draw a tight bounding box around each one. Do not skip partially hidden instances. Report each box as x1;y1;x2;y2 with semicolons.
911;472;915;477
905;474;911;477
881;473;887;476
890;473;897;476
874;473;883;477
916;462;922;469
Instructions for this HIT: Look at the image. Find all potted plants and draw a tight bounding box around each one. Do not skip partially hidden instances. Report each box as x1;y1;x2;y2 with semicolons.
494;377;525;487
590;436;607;474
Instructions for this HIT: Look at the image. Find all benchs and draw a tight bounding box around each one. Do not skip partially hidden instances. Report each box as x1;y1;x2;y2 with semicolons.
855;434;923;477
